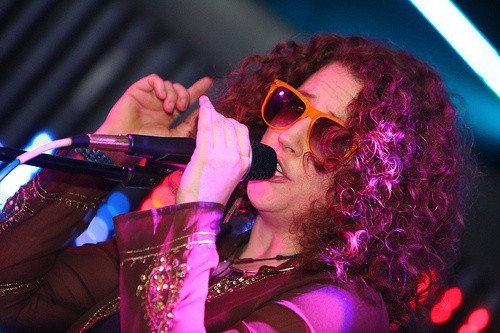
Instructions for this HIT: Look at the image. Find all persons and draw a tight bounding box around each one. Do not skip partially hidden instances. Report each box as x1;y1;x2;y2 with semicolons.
0;36;473;332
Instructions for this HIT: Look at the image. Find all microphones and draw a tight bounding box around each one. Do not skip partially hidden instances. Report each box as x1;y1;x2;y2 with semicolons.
71;133;277;182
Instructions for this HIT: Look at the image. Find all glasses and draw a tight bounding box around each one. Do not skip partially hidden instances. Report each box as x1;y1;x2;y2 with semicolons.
260;79;364;172
